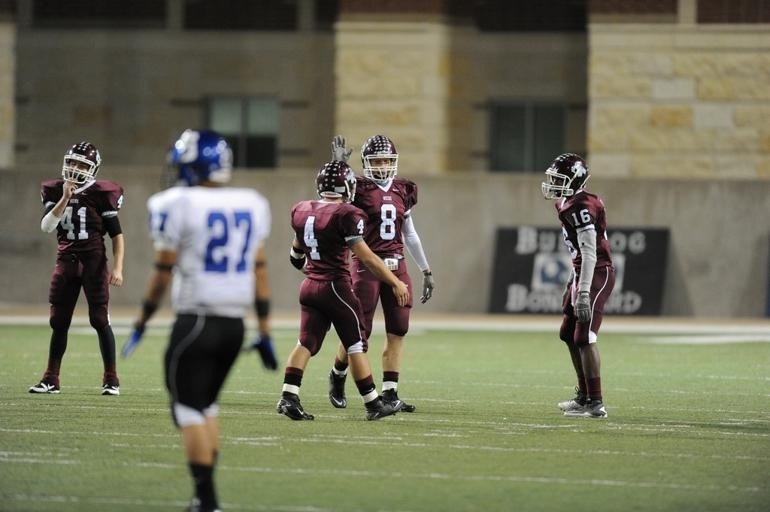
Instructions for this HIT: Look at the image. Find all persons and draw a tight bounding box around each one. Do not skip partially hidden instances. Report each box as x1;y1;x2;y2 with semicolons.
27;140;126;396
118;127;280;512
328;134;434;412
276;160;410;421
542;152;615;418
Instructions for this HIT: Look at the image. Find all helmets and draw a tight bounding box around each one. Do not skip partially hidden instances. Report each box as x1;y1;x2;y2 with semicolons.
541;152;590;200
315;161;357;203
62;141;102;184
361;135;399;184
170;127;235;186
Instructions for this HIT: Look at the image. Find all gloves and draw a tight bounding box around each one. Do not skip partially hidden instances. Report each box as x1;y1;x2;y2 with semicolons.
420;269;435;303
121;321;144;359
330;135;353;163
248;334;278;371
574;292;592;321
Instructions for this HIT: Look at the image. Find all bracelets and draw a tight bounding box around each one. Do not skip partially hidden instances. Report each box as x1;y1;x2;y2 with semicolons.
254;297;271;321
577;293;590;296
424;271;433;276
141;296;158;318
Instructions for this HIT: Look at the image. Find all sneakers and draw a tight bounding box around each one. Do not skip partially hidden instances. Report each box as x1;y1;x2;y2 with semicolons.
276;392;314;420
559;386;586;411
102;383;121;395
564;400;607;418
328;369;347;408
366;395;403;421
383;390;415;412
29;380;61;394
